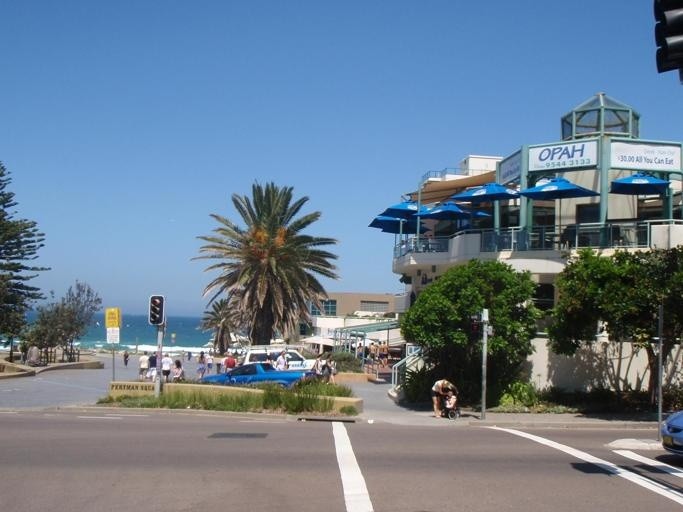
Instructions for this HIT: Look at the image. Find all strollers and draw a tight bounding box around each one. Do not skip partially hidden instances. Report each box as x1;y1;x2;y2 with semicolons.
439;387;460;420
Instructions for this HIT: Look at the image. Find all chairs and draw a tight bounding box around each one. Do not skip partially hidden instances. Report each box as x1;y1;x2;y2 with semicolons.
552;223;577;249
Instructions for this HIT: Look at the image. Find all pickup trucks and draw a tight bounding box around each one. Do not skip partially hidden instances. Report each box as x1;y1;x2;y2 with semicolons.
202;362;314;388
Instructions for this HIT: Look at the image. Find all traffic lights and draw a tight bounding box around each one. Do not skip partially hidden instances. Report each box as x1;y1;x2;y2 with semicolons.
471;322;479;332
654;0;683;72
148;295;165;326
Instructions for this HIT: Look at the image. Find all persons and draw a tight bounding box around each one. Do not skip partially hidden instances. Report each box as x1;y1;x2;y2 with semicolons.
432;380;457;418
138;342;389;386
124;350;129;368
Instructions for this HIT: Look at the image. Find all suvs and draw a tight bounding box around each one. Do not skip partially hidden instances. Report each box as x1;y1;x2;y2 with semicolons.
242;349;337;375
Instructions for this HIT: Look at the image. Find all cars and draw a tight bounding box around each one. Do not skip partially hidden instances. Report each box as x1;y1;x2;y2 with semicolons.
661;409;683;456
